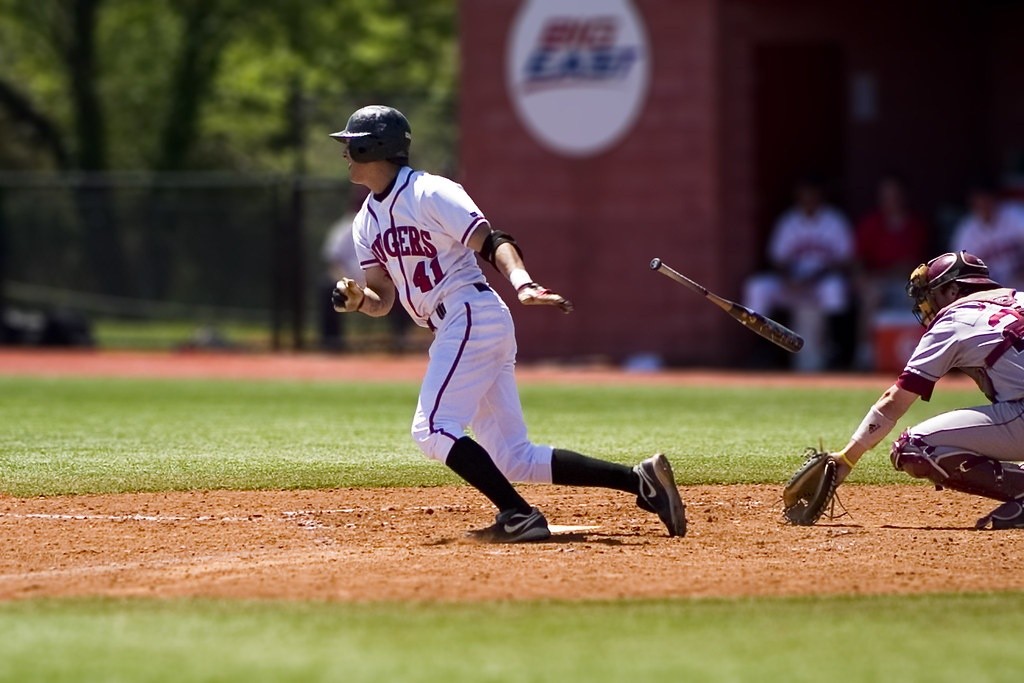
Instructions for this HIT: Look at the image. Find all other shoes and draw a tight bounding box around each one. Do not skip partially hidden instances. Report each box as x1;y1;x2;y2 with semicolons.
976;494;1024;528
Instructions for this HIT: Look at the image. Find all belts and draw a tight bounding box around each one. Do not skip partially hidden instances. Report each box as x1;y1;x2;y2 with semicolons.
426;282;494;332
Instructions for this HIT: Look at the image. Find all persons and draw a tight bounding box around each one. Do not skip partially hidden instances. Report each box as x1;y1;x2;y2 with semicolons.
854;178;941;376
783;250;1024;531
331;106;686;542
953;182;1024;291
742;183;852;376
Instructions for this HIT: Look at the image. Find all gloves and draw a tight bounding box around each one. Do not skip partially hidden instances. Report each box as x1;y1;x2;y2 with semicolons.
331;277;364;312
516;282;573;314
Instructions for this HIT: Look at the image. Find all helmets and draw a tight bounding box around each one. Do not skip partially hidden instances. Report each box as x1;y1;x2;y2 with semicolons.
926;252;1002;292
328;105;411;164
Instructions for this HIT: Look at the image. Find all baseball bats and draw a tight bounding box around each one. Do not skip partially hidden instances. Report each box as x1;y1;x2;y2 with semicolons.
648;258;807;354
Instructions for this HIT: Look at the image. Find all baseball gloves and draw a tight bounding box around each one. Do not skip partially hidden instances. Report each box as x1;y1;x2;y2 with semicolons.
779;437;856;528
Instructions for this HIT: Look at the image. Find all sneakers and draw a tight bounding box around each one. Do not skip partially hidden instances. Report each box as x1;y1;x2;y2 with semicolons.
463;506;551;545
632;453;686;537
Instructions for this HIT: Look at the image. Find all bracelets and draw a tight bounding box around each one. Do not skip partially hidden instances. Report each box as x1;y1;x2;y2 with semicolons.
510;268;533;291
851;404;897;450
839;452;855;469
